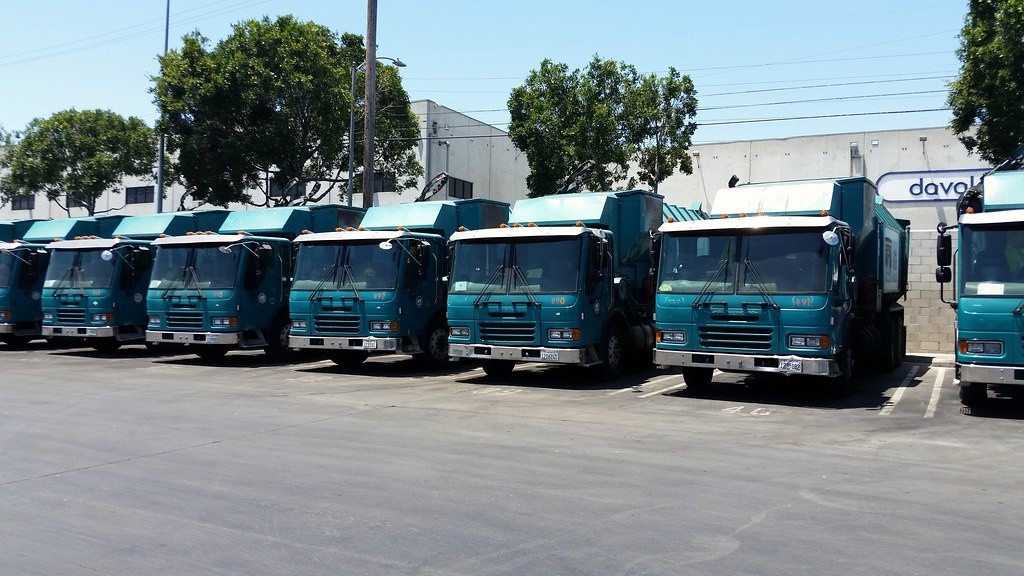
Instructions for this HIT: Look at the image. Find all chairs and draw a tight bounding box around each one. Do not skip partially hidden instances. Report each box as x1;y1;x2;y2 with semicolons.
968;249;1011;283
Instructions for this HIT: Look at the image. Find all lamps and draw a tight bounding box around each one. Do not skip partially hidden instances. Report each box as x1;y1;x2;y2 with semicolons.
920;136;928;155
693;151;700;168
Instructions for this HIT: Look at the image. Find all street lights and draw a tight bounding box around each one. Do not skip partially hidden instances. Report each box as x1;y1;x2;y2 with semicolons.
348;57;407;207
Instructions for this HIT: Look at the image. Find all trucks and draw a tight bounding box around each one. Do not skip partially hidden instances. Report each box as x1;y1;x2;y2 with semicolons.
648;176;911;399
288;197;512;373
446;189;711;382
41;209;237;351
146;204;366;361
0;215;136;344
936;169;1024;401
